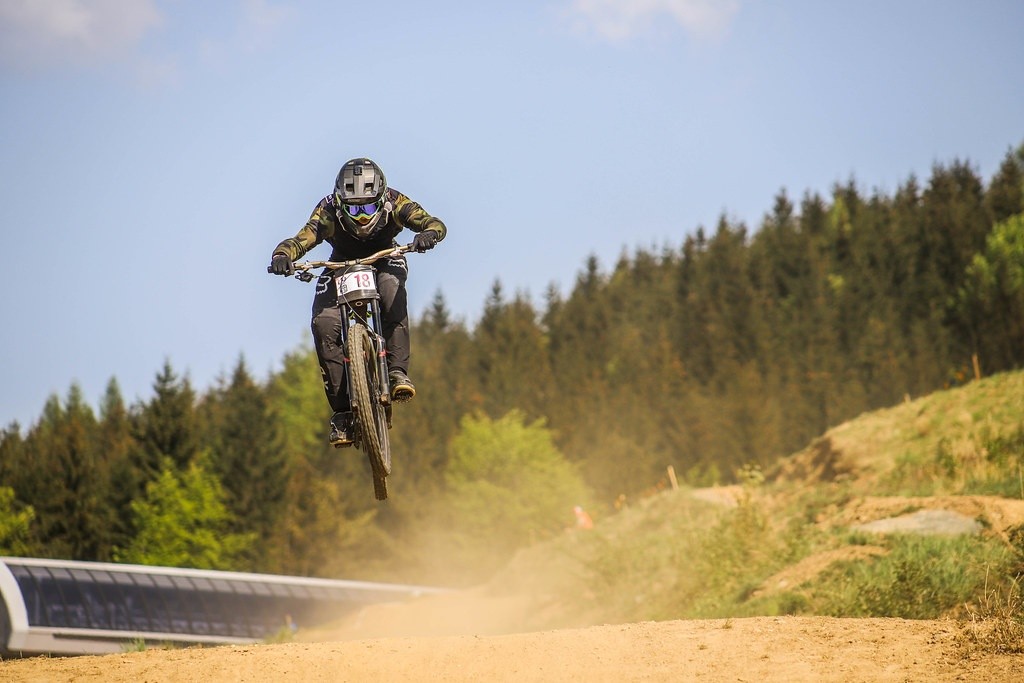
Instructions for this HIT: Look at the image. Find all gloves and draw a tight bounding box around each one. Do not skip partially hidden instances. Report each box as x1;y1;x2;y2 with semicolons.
411;230;437;254
271;252;296;277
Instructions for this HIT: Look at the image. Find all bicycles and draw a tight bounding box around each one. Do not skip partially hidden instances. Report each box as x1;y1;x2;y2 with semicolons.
267;238;437;500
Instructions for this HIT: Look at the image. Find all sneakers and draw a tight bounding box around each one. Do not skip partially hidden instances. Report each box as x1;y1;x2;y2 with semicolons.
329;410;356;444
389;370;416;404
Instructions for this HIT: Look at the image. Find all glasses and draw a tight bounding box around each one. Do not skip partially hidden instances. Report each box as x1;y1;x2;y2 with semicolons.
334;193;387;220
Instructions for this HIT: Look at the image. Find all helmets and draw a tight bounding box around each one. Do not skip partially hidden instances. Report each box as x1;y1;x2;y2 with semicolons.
334;158;387;236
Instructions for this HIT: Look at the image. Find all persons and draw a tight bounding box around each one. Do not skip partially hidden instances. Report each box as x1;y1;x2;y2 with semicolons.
271;157;448;445
